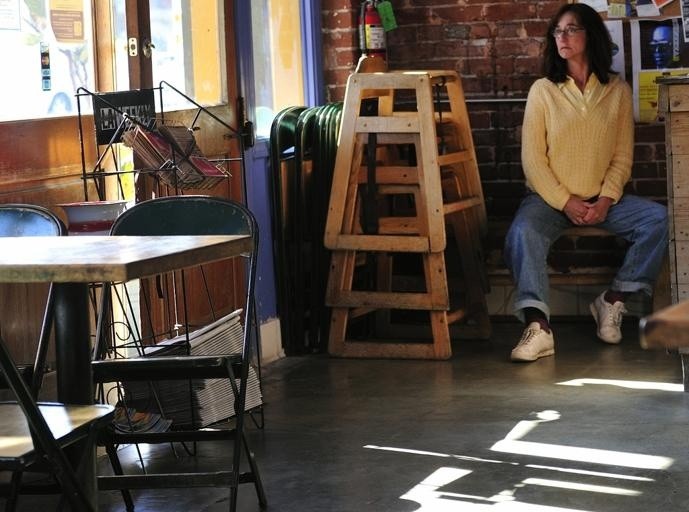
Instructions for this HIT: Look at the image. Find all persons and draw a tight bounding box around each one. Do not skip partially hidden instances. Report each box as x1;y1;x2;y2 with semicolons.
645;25;674;69
499;3;670;365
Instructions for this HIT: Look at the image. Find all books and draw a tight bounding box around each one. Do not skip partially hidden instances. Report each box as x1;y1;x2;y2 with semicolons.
111;404;175;437
119;124;226;191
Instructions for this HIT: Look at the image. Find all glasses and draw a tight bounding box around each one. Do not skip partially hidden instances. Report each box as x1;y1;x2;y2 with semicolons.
649;40;674;53
552;27;584;37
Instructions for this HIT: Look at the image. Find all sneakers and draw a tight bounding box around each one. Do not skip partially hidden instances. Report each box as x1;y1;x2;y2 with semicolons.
510;322;554;362
590;290;628;345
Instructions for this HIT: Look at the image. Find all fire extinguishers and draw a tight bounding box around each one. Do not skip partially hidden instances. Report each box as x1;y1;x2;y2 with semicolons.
359;0;398;71
